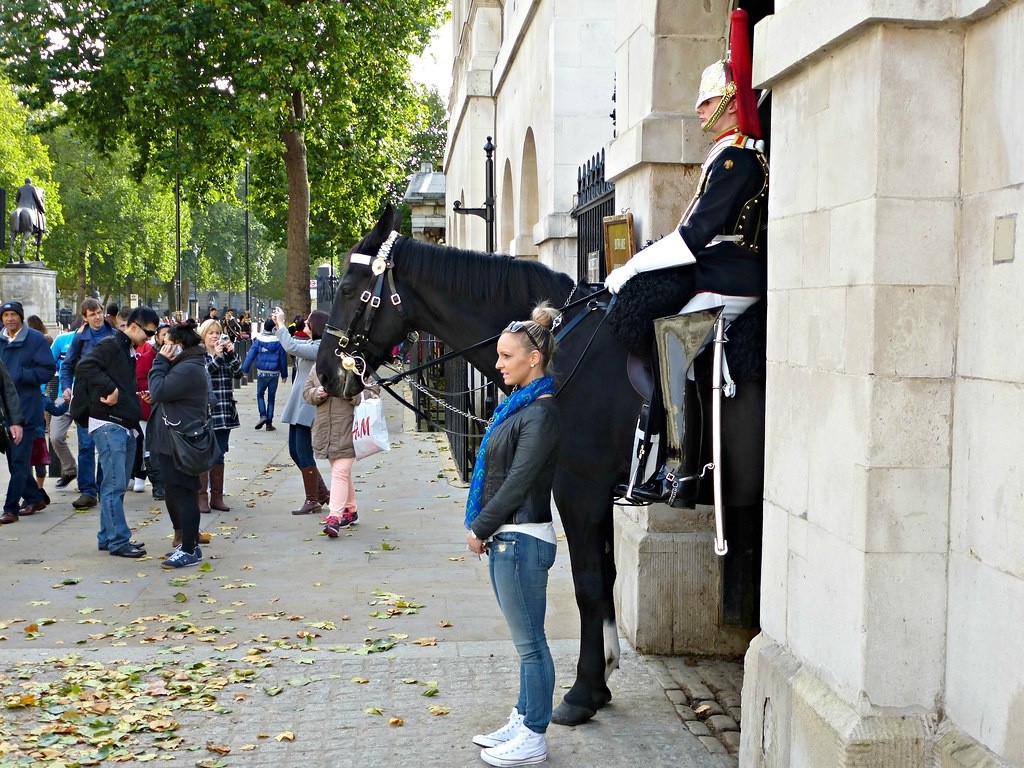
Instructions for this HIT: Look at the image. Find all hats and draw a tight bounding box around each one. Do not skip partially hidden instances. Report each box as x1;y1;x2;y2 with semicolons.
0;301;24;321
263;317;277;335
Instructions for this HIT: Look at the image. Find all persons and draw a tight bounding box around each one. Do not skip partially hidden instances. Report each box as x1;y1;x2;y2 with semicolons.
271;306;330;515
603;60;769;510
242;318;288;431
0;298;252;525
15;177;46;215
464;300;564;767
71;307;159;558
302;362;380;538
136;319;220;570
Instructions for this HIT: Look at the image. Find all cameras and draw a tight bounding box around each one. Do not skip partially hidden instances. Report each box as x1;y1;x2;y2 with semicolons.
218;334;229;344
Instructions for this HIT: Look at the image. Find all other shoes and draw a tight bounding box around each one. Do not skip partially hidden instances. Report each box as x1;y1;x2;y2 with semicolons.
254;416;277;431
127;475;145;492
20;487;51;510
72;495;98;511
173;534;210;547
55;474;77;489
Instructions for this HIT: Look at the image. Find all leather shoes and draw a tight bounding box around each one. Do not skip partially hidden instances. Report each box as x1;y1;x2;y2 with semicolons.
153;484;166;500
98;542;146;558
0;500;47;524
613;465;699;510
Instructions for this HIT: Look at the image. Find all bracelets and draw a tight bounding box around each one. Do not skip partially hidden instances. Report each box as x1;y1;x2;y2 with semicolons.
469;530;479;540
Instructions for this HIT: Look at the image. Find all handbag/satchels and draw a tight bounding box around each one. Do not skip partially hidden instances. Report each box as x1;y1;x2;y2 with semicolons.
31;438;52;466
236;331;250;341
162;412;222;477
351;388;392;462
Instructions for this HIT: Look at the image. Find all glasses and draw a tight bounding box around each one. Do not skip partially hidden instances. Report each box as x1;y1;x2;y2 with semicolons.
505;320;541;352
128;320;155;337
156;322;170;331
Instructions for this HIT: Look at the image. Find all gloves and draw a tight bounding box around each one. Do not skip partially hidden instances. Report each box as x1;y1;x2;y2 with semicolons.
604;229;697;295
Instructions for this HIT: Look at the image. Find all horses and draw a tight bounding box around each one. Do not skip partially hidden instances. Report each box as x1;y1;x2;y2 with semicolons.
315;204;768;726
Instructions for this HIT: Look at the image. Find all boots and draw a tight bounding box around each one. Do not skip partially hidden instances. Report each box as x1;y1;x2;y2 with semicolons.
197;462;230;513
292;465;330;515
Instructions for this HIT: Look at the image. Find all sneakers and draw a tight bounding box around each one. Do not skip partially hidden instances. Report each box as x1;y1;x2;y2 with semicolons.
160;543;204;571
471;707;547;768
320;507;360;538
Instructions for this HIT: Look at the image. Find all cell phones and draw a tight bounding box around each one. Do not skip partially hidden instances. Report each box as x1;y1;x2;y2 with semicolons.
169;345;183;357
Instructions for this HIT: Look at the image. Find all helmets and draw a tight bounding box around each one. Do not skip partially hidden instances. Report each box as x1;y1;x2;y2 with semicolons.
694;8;761;139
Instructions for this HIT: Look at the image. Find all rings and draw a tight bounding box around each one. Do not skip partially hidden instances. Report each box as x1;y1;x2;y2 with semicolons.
466;545;471;550
317;396;321;399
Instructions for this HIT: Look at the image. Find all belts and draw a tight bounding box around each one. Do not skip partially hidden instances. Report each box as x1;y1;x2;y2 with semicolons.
713;234;743;242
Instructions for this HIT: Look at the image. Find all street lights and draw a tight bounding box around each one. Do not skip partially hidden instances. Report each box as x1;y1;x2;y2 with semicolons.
143;235;151;307
225;250;232;315
193;243;201;326
254;257;263;334
